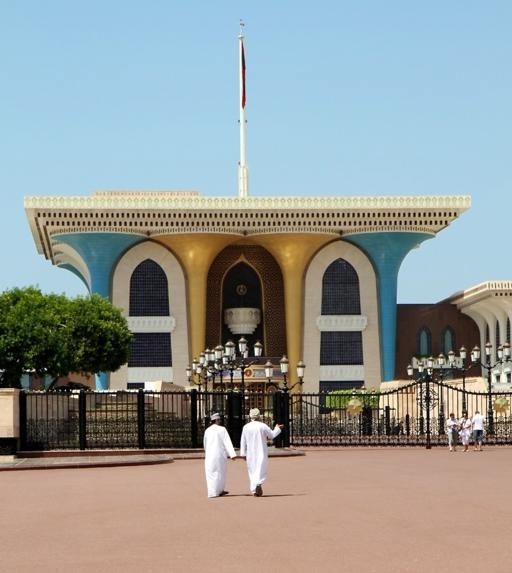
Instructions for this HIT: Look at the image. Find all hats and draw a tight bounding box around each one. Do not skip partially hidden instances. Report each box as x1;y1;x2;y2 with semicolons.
249;408;260;419
211;413;220;421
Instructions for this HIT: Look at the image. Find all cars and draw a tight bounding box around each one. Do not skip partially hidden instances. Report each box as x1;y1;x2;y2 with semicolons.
50;382;92;391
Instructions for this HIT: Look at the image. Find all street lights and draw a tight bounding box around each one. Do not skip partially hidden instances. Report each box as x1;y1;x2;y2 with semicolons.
186;336;305;444
405;341;510;448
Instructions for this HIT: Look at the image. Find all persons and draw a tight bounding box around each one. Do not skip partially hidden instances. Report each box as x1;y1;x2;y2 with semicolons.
203;413;237;498
448;409;489;453
239;407;285;497
